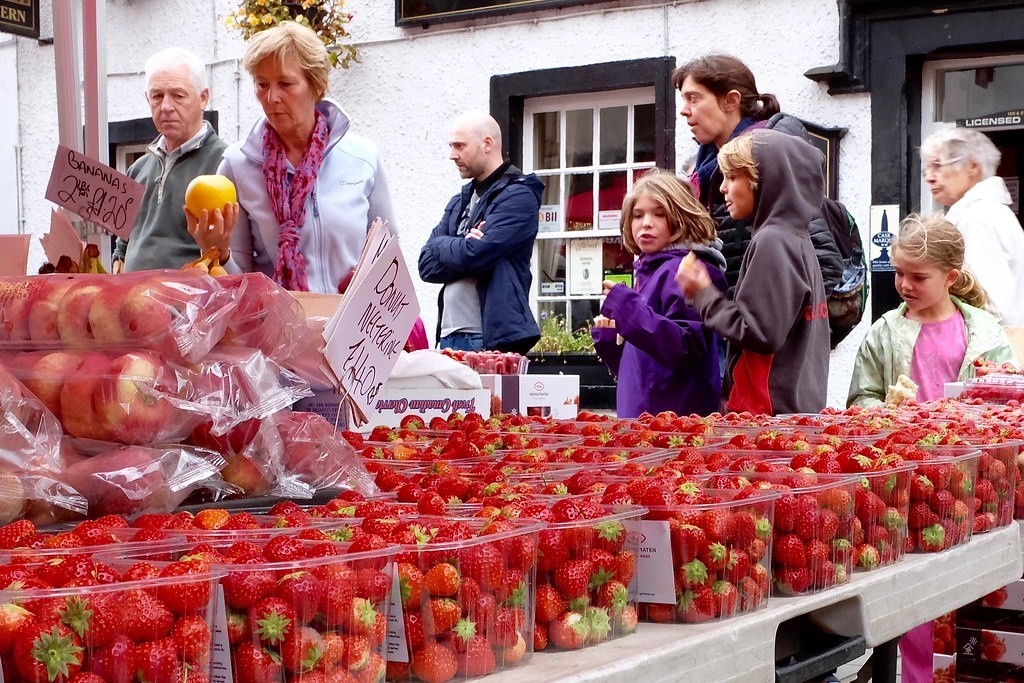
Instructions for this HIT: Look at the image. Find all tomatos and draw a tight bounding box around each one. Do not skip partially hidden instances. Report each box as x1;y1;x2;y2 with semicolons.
963;374;1024;407
437;347;529;374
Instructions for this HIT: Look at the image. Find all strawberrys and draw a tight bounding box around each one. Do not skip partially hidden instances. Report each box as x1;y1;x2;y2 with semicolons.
972;358;1024;377
1;398;1024;682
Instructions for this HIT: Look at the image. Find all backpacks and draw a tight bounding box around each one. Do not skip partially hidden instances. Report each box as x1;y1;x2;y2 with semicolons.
820;198;868;348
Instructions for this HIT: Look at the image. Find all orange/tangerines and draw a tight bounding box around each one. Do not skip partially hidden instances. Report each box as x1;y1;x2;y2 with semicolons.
185;174;236;224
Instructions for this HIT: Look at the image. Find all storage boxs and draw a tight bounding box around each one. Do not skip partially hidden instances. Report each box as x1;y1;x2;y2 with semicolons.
3;376;1024;681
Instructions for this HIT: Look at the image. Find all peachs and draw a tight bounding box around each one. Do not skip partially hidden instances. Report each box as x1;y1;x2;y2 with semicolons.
1;273;366;524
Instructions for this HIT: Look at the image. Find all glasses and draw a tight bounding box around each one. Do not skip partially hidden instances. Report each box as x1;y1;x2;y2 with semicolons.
923;156;965;172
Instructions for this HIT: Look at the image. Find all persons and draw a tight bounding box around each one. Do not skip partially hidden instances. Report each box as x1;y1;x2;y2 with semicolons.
112;47;230;271
845;211;1019;682
183;20;400;293
673;127;832;414
591;167;729;418
672;52;868;389
920;127;1024;344
417;110;544;354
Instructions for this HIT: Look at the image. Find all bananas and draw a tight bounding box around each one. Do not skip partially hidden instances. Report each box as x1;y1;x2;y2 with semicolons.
54;244;230;278
